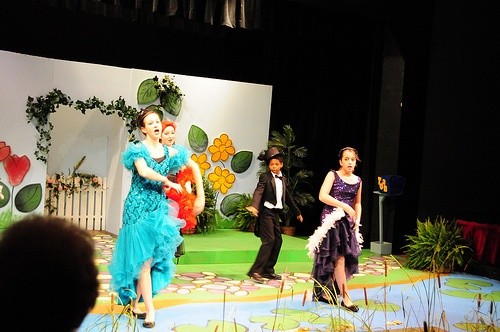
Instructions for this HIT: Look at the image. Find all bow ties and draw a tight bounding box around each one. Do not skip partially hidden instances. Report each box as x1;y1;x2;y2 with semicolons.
275;175;284;180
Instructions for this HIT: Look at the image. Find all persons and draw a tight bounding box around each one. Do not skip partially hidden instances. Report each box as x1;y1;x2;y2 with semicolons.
312;147;362;311
0;216;99;332
246;148;303;282
108;110;185;328
160;121;205;255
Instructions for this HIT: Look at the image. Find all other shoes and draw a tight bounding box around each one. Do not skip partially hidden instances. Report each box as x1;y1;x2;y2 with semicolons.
143;322;154;328
319;296;334;304
127;308;145;319
340;300;359;312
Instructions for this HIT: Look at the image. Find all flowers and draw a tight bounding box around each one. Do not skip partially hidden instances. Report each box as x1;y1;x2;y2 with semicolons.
46;156;101;213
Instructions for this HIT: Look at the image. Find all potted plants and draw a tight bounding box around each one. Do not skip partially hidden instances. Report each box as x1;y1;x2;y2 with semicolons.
402;215;472;272
260;125;315;236
180;176;217;235
226;193;256;231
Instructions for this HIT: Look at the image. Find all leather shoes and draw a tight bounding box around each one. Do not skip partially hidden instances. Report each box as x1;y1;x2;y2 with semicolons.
264;273;282;280
247;272;262;281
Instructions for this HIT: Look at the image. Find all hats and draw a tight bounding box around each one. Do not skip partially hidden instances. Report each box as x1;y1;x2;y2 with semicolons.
263;147;285;166
